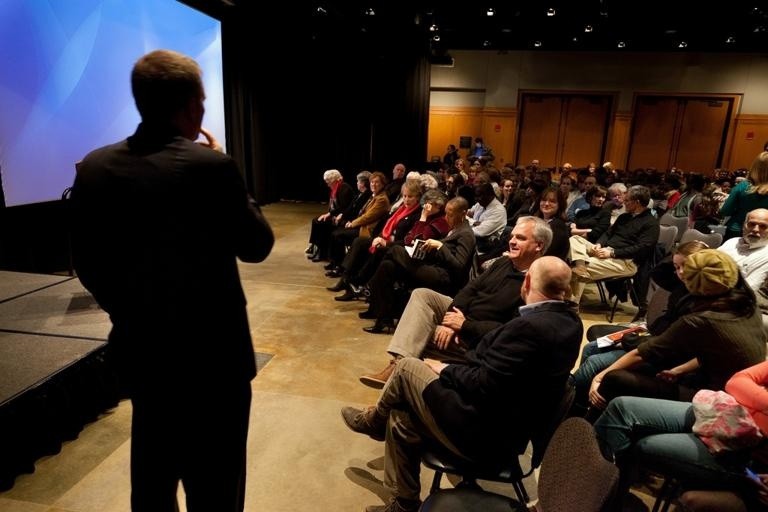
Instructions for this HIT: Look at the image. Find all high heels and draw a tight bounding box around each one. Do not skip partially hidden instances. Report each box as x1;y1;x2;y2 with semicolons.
361;322;395;334
335;291;361;302
326;280;350;291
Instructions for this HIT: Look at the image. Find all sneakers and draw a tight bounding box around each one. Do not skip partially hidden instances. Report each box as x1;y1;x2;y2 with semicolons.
571;266;593;282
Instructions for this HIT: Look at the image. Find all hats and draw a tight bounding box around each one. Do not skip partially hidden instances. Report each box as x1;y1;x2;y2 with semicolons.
681;247;739;297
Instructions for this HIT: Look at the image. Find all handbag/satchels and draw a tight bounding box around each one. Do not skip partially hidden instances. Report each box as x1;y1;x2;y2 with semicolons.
692;387;763;454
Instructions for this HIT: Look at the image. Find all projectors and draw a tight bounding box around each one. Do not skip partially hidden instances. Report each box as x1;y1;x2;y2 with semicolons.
427;53;455;69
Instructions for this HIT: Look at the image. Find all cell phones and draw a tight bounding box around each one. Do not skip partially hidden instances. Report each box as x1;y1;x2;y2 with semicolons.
744;466;767;492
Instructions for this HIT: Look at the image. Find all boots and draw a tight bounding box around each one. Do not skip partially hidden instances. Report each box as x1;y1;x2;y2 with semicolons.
338;401;387;443
358;359;398;389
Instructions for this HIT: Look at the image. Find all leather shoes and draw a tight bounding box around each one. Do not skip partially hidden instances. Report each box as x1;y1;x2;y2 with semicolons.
325;270;342;277
367;497;419;511
324;263;338;269
358;311;376;319
312;256;326;262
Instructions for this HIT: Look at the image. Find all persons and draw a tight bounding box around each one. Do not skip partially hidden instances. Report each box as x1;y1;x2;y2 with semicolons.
65;49;275;510
303;133;768;512
